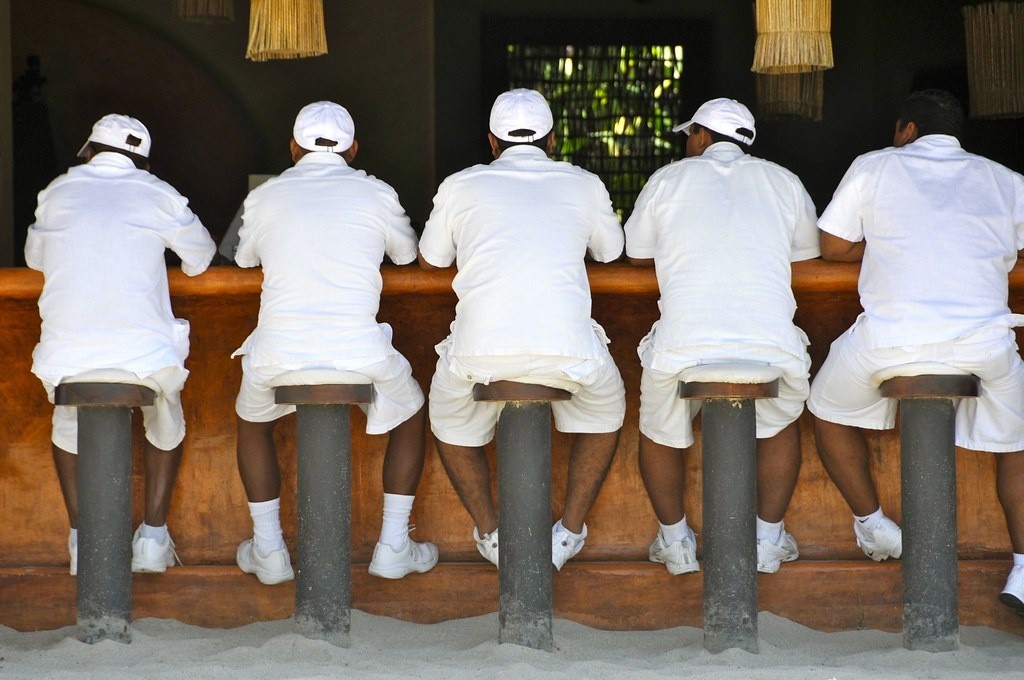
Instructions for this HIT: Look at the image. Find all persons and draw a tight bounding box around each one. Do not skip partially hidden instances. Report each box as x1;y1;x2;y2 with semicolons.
228;102;438;586
803;84;1023;615
621;96;823;574
22;113;216;577
415;88;626;572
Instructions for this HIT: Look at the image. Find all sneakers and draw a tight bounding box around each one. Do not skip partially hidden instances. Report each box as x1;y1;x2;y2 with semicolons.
552;519;585;573
854;516;902;562
132;523;183;573
473;526;499;569
999;566;1024;617
756;522;799;573
368;527;439;579
649;526;700;575
68;536;78;575
237;534;295;585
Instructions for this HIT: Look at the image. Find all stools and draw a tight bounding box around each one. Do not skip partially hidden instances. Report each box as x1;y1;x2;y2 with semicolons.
473;377;582;651
267;369;375;647
678;363;781;654
870;362;981;653
54;368;161;645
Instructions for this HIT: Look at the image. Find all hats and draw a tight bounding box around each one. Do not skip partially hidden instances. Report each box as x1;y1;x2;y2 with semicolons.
77;113;151;157
490;88;553;142
672;98;757;146
293;100;355;152
